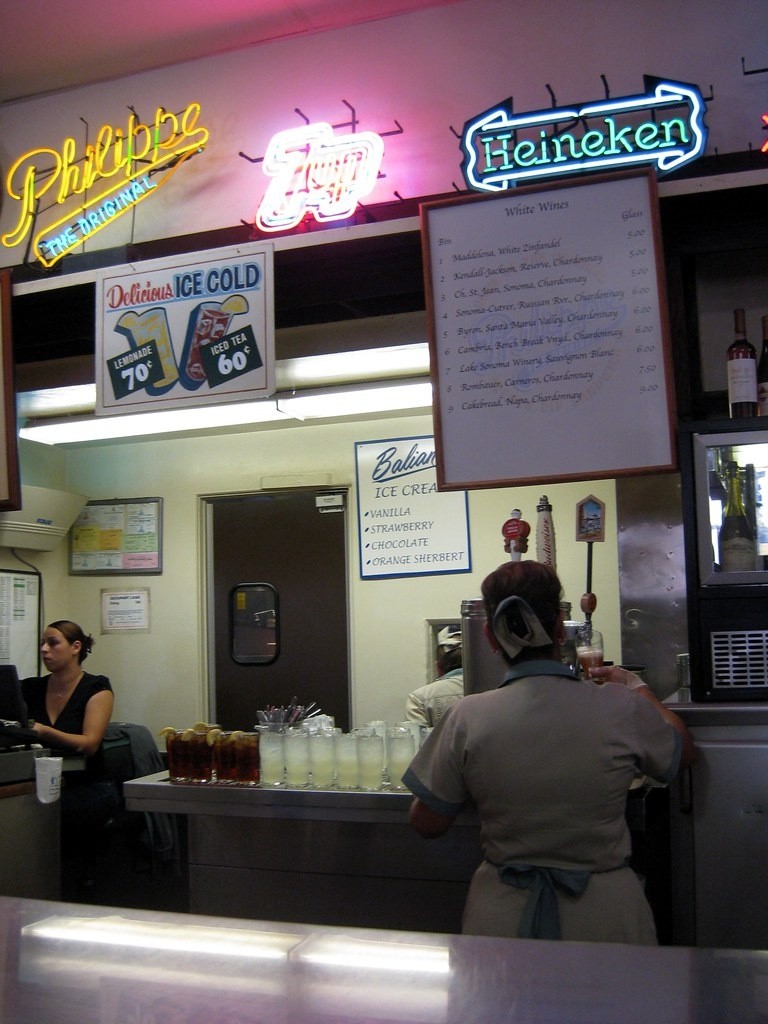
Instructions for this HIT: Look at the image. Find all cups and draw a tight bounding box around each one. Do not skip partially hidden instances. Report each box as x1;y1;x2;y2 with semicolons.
574;629;605;681
326;714;342;735
166;724;259;783
420;728;435;747
387;734;414;790
309;732;334;788
259;732;285;786
358;735;384;789
334;734;358;789
285;732;309;788
352;720;419;734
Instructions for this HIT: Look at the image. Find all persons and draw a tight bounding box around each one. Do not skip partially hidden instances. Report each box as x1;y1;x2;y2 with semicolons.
16;620;116;902
401;562;690;948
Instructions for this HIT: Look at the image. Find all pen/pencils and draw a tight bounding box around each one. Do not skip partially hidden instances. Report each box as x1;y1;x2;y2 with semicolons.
256;695;322;724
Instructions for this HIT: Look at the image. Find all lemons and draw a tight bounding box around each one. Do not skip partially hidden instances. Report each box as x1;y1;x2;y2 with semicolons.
181;729;198;741
206;729;224;745
159;727;177;739
192;722;209;733
229;731;246;740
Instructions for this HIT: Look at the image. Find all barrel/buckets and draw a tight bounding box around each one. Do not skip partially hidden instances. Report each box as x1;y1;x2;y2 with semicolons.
461;598;571;696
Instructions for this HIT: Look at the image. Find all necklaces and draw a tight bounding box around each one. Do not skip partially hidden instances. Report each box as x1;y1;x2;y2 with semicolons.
49;676;77;699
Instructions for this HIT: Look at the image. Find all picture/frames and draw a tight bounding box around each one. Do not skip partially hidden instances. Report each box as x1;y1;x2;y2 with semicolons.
1;269;21;512
418;162;677;492
69;497;164;575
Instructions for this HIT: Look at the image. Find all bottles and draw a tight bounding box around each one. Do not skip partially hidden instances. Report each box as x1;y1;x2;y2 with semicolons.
743;463;757;544
726;308;759;420
759;315;768;420
718;460;758;571
